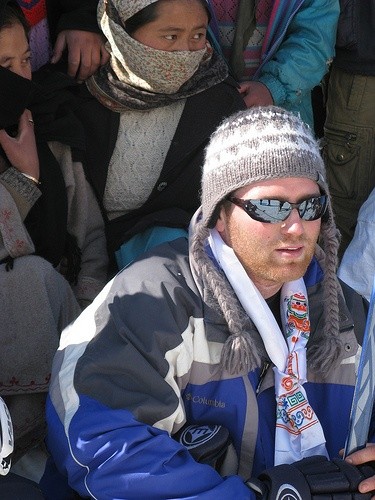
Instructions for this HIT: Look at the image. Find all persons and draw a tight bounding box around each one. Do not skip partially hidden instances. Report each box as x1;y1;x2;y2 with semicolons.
0;0;115;500
337;185;375;303
16;0;110;86
320;0;375;272
46;104;375;500
57;0;244;266
206;0;341;143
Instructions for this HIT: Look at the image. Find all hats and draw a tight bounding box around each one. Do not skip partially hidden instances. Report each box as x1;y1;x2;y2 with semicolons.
190;106;342;373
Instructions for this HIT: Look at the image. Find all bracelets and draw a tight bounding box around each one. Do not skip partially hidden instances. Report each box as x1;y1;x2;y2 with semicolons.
20;171;42;187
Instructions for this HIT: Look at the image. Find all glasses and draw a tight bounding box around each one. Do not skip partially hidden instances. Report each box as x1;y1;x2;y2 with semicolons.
225;193;329;223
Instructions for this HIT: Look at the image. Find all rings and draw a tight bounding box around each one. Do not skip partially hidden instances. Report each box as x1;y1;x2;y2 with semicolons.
28;117;34;123
